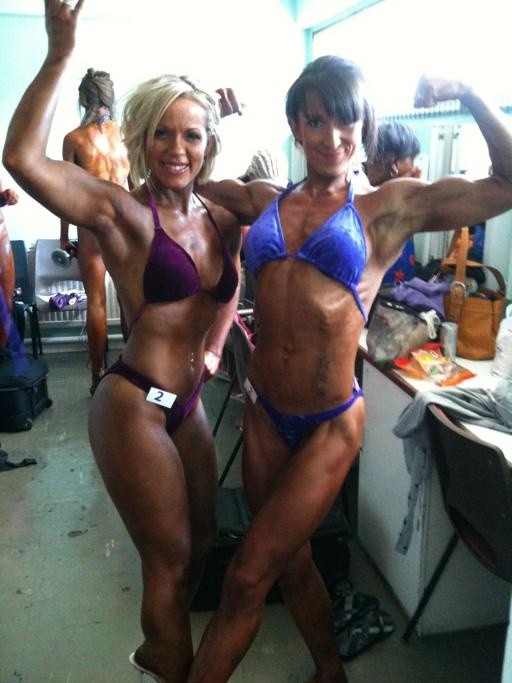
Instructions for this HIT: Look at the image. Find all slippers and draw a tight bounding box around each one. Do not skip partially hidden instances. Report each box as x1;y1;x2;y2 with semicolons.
332;593;397;661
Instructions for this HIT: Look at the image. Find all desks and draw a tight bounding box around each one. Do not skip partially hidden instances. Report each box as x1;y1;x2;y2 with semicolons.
357;327;512;682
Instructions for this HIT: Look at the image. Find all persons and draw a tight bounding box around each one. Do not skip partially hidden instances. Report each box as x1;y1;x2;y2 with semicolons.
0;183;17;353
59;66;143;400
352;121;420;185
183;53;511;682
1;0;245;682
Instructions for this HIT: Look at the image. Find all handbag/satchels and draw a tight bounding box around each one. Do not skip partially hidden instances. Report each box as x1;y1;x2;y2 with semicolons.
367;282;441;362
445;283;504;360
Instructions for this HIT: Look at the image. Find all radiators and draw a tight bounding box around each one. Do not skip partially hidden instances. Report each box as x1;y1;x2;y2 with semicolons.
24;269;121;328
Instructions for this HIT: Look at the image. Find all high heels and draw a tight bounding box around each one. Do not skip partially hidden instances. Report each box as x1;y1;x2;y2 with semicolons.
129;652;165;683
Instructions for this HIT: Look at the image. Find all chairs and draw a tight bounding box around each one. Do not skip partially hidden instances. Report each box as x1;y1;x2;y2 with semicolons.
10;239;109;369
212;312;255;489
403;404;512;648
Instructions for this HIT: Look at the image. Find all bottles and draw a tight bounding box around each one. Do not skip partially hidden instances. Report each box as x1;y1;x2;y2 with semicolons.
375;308;442;367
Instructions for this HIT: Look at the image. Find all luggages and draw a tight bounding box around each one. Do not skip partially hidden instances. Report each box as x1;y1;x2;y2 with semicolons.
187;484;352;613
2;357;52;432
